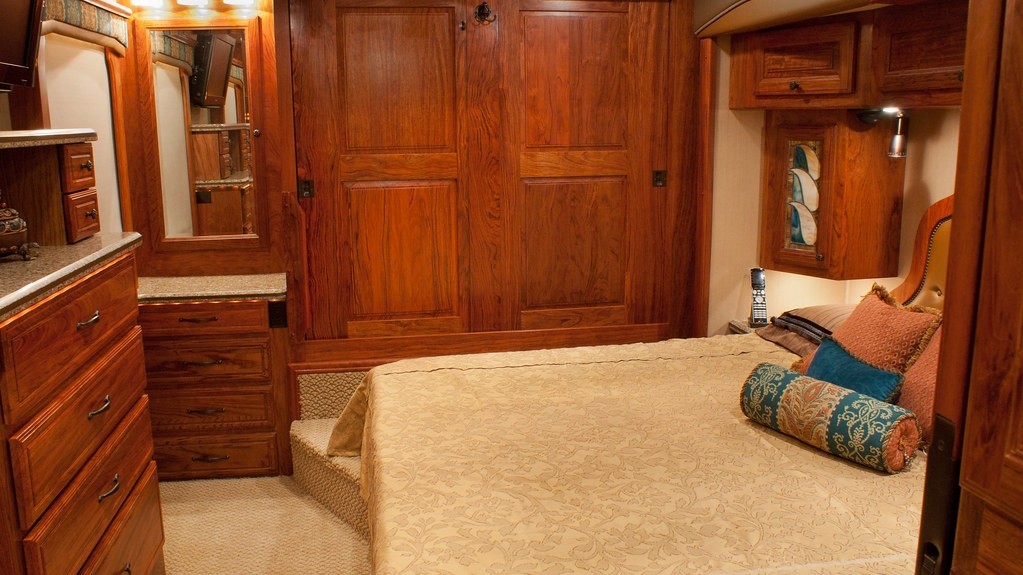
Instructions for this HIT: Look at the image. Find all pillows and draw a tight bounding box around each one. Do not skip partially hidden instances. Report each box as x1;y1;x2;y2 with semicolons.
739;362;922;475
803;334;906;403
791;282;942;373
754;302;858;357
897;315;942;450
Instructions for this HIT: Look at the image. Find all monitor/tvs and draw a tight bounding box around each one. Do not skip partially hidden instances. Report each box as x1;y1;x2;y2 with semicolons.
0;0;44;87
189;31;236;108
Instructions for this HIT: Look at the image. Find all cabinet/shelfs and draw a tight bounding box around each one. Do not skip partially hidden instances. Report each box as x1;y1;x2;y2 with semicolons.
0;232;167;575
726;0;971;110
759;110;904;280
189;122;251;177
2;126;103;246
137;273;305;480
193;181;256;234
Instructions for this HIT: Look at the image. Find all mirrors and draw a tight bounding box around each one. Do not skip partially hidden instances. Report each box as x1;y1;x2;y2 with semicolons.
133;14;274;253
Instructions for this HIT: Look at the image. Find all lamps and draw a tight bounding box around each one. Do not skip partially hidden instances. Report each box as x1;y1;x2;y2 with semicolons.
850;108;908;158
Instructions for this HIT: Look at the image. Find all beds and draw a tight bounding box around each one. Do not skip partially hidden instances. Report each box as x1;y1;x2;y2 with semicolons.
326;195;955;575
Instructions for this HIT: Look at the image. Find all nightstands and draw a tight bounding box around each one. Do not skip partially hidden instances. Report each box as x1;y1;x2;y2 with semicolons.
729;319;773;335
